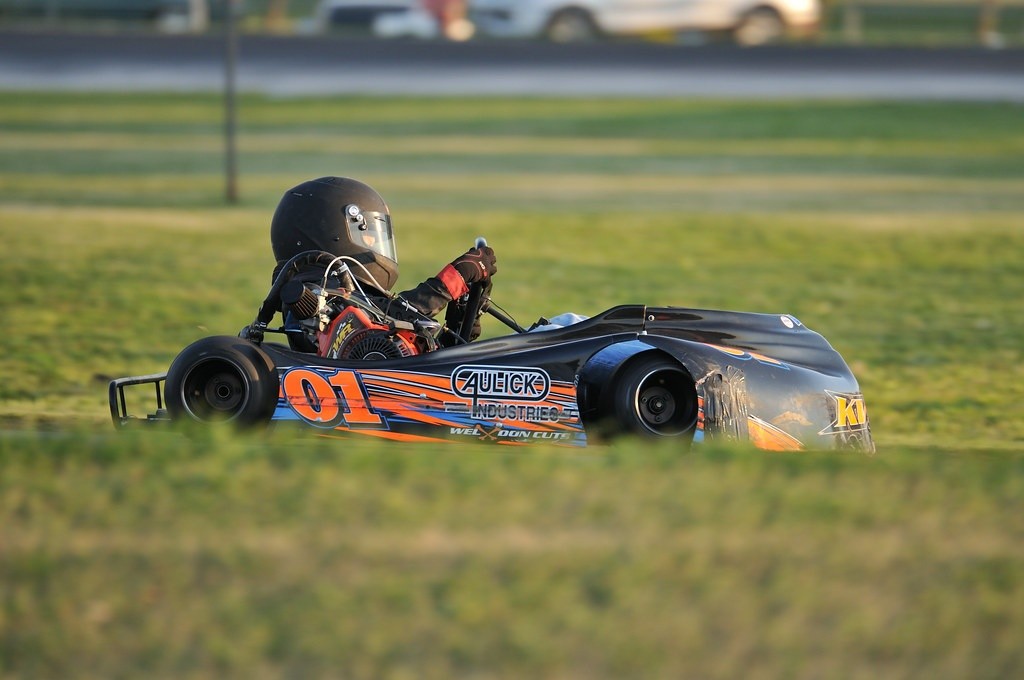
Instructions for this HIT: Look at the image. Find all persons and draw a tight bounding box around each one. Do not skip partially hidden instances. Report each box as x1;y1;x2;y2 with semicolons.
270;176;498;360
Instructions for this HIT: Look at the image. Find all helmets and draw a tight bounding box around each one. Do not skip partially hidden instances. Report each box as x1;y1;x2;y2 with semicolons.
270;177;399;292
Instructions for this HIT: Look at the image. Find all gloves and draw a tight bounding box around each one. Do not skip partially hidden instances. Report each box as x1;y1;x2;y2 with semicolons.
450;247;497;289
436;299;481;348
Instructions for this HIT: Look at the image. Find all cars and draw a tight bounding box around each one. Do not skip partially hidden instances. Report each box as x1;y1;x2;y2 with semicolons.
292;0;825;46
